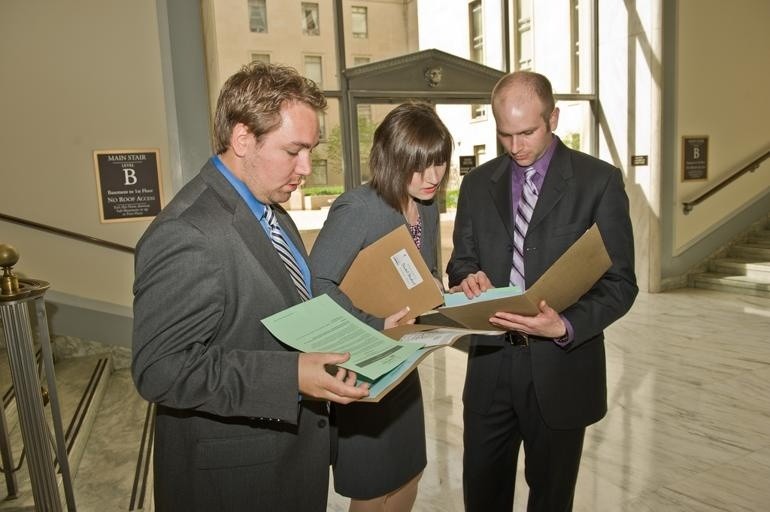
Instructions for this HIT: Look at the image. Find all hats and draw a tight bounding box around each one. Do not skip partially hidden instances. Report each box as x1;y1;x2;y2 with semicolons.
264;204;312;308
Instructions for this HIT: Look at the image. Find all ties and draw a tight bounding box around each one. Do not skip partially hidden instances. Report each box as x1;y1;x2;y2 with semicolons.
507;162;540;293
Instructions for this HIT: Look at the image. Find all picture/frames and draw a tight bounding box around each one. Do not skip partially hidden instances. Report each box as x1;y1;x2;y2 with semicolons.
92;148;166;225
679;136;709;184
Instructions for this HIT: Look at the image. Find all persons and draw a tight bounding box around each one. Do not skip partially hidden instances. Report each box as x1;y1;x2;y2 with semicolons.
308;99;454;512
445;70;641;512
130;59;372;512
